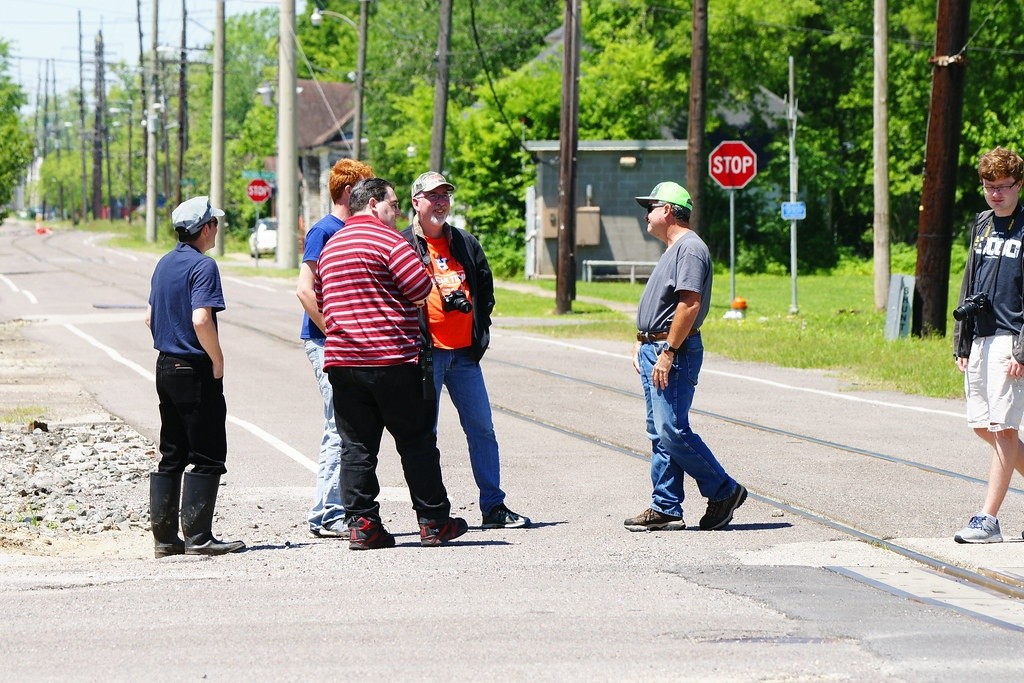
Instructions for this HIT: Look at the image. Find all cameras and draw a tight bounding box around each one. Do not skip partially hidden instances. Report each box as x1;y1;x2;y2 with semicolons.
441;290;472;313
952;292;992;321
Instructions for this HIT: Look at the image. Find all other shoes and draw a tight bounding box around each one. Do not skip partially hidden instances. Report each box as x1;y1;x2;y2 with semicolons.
311;520;350;538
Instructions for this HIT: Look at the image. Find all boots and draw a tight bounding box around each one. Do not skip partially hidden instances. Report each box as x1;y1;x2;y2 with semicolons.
149;469;186;559
180;473;246;555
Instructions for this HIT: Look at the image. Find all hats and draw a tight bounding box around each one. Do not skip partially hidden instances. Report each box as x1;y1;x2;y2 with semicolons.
411;170;455;200
172;195;226;236
635;181;693;213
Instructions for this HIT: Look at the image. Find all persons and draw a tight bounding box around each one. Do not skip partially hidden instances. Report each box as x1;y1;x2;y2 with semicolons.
621;182;749;532
953;148;1023;543
294;158;374;539
142;196;247;558
398;171;531;529
315;177;469;550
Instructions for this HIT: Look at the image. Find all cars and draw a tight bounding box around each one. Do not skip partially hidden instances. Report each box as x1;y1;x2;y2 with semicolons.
247;218;277;258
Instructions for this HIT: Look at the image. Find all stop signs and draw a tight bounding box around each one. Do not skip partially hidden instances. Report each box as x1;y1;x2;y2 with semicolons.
247;179;271;204
708;142;757;190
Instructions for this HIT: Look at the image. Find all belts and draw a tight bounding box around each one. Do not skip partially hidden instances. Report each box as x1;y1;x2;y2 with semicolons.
636;328;701;341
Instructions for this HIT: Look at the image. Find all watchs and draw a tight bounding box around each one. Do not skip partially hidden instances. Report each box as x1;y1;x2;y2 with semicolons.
662;343;679;355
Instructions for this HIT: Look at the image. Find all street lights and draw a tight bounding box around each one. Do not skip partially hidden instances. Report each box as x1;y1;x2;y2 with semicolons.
109;108;133;226
157;46;225;258
310;7;366;160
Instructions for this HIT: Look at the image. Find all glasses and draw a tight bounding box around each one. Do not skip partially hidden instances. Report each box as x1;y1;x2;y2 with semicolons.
416;192;454;201
982;172;1023;193
647;202;673;213
197;218;218;228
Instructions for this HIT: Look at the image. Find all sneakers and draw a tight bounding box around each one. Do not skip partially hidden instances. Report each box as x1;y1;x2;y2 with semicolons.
420;517;468;546
699;482;747;530
481;503;530;528
953;513;1005;544
348;514;396;549
623;508;685;530
